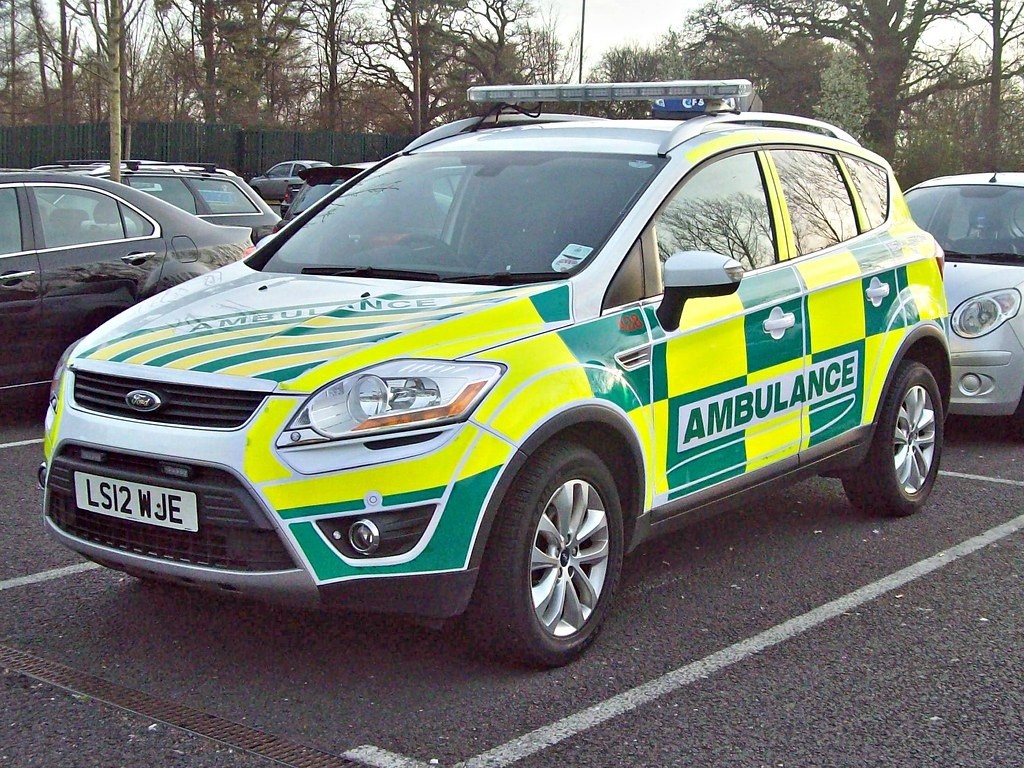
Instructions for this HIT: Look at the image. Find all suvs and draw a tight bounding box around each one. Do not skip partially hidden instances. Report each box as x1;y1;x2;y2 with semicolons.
32;161;283;249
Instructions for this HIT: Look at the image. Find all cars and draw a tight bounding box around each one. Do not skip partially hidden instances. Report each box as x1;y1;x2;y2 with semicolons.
901;173;1024;416
0;169;267;426
281;183;304;218
273;163;465;252
249;161;332;201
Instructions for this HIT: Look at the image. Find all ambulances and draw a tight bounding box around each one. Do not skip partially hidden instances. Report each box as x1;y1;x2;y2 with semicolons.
36;78;952;673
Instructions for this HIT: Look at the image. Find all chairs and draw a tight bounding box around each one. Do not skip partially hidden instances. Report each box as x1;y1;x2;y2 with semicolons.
952;202;1017;253
84;203;119;241
